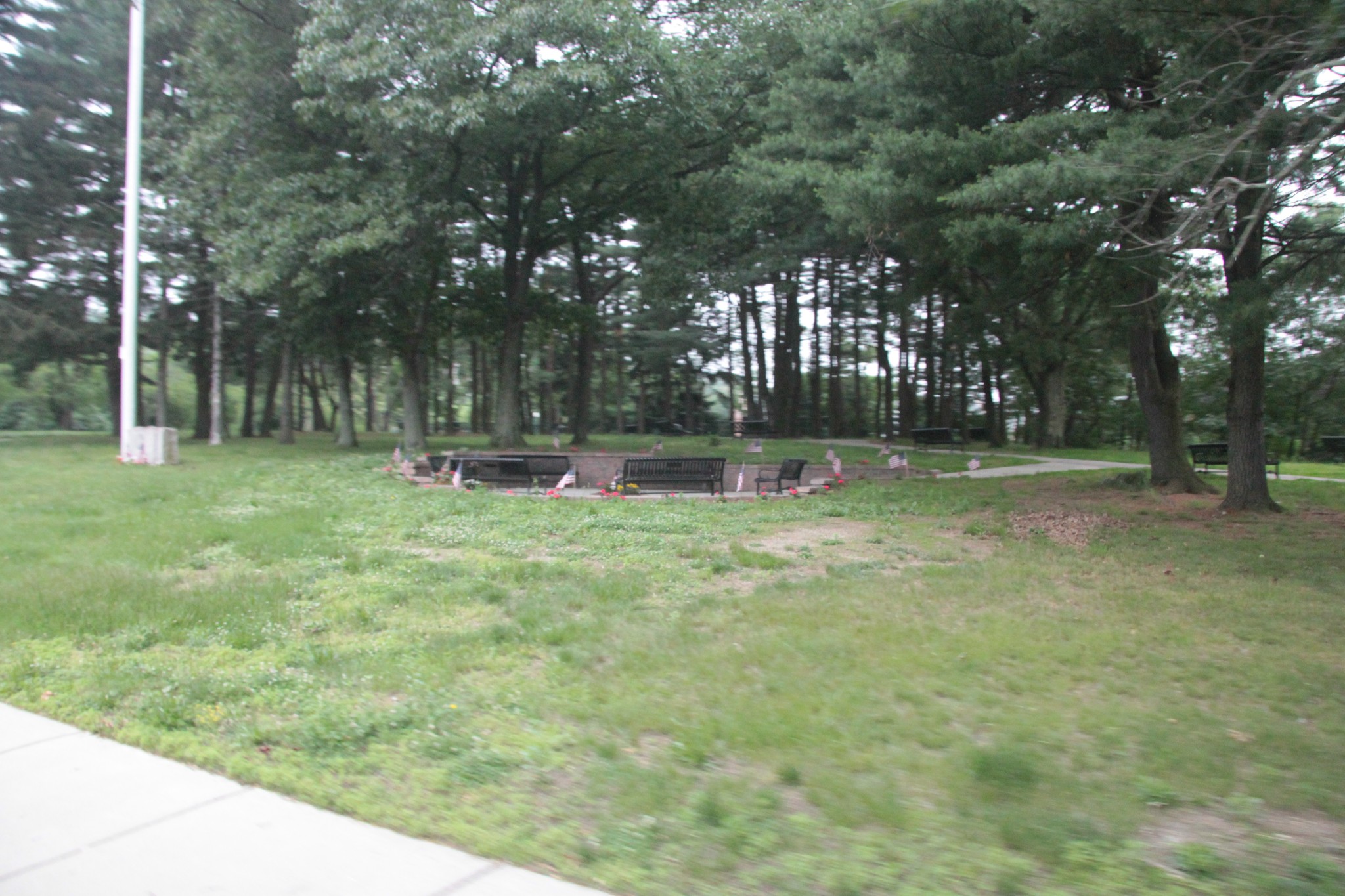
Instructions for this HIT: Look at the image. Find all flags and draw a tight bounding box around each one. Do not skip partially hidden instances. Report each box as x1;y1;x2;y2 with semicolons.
878;444;890;456
392;438;842;492
968;455;980;470
887;451;908;469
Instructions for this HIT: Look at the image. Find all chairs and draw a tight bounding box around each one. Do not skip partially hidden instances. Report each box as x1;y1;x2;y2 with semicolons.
753;458;809;494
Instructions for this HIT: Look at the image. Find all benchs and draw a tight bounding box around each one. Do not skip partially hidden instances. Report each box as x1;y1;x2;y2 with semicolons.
496;455;576;488
728;419;785;440
448;457;548;496
620;454;727;497
909;426;959;450
1319;433;1345;461
1186;440;1282;481
